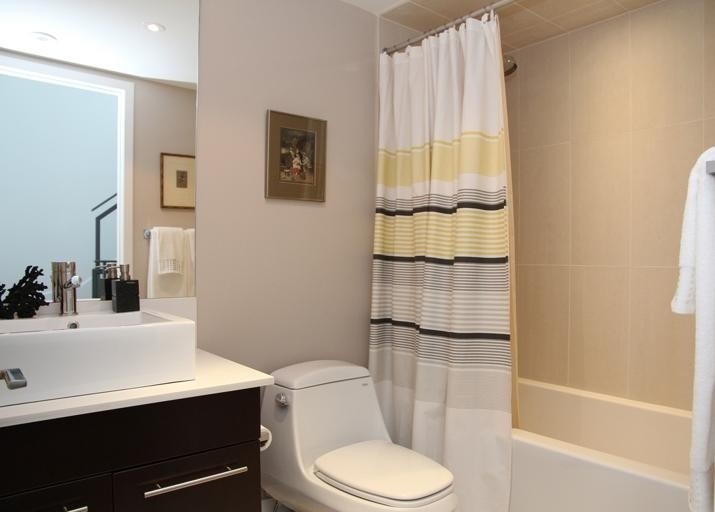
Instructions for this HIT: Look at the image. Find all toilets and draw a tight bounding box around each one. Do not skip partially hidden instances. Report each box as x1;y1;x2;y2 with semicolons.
259;358;456;512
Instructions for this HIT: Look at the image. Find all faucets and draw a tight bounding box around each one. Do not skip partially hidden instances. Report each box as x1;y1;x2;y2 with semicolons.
50;262;62;302
58;261;82;315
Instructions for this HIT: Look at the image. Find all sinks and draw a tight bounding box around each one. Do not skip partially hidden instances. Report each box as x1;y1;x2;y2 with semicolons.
0;309;199;411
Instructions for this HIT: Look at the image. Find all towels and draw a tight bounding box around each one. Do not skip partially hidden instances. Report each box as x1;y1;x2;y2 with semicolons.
145;225;194;298
670;148;715;512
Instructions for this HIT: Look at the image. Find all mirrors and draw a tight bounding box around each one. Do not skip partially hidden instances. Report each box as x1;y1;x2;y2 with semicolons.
0;47;197;299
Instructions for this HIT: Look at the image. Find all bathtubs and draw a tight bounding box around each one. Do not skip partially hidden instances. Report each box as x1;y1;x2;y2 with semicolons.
511;376;693;512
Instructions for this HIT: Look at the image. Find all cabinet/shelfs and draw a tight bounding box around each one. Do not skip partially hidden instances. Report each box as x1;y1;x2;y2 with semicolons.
0;350;277;509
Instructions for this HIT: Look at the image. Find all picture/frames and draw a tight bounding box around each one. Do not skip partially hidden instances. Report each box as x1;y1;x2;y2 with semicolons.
265;109;327;203
159;152;195;211
